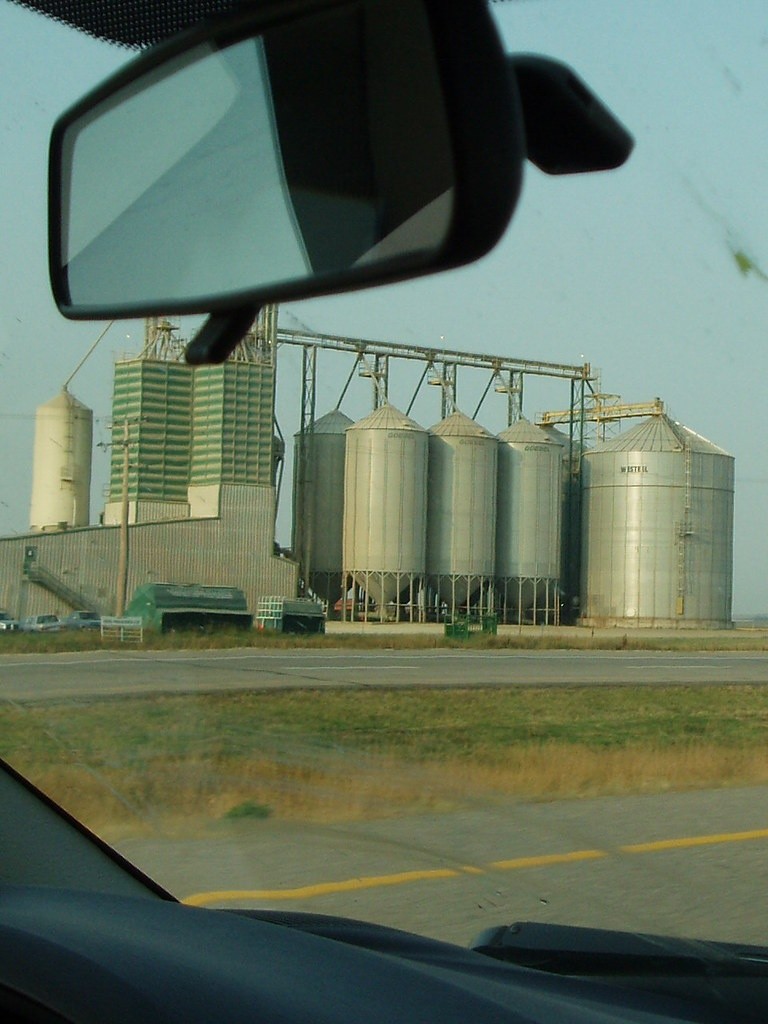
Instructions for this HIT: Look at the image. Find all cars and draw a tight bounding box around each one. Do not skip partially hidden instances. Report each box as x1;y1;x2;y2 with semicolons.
65;611;101;630
23;615;65;631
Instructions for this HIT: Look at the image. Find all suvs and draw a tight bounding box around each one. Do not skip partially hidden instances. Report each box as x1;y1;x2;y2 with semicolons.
0;609;20;631
